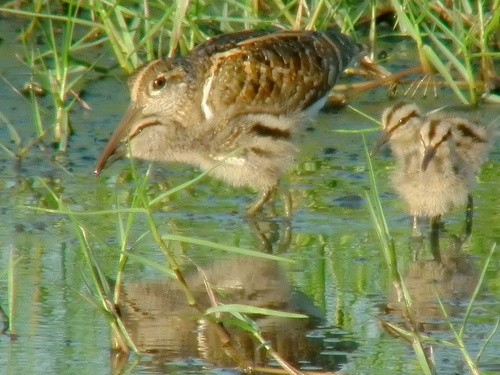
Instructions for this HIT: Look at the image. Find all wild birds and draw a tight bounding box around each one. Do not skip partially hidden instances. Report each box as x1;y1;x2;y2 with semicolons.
93;28;488;219
108;219;480;375
